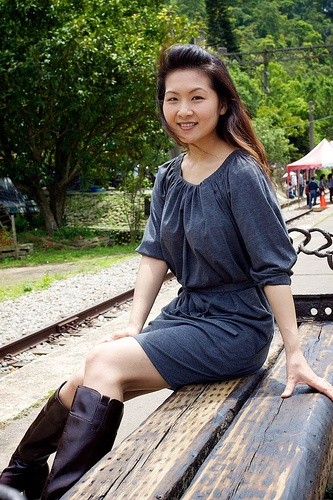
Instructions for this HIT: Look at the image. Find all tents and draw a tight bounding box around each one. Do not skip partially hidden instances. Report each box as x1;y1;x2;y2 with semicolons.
285;138;333;211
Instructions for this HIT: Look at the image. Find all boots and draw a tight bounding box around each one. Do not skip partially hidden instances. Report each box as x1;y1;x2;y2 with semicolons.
0;382;69;500
40;384;124;500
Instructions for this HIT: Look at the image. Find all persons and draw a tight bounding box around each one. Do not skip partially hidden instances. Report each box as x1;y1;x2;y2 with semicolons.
327;174;333;202
0;45;333;500
299;171;304;197
284;173;297;198
319;174;326;193
308;177;318;205
305;180;309;205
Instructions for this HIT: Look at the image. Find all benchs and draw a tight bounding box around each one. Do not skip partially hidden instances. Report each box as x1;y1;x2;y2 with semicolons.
58;227;333;500
